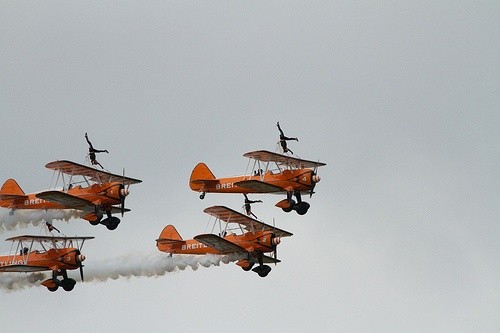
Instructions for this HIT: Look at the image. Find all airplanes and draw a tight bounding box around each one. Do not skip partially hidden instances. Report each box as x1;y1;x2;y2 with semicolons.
0;159;142;230
155;205;294;278
0;234;96;292
189;150;327;216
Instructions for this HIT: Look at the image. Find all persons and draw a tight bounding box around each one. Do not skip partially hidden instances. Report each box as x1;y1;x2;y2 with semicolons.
68;184;73;190
45;221;60;233
85;133;108;169
222;231;227;238
20;247;29;255
256;169;264;176
244;193;263;218
276;122;298;154
